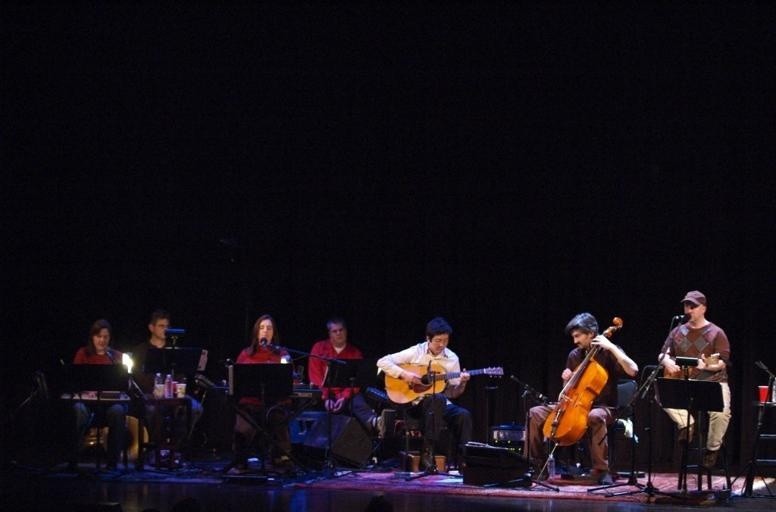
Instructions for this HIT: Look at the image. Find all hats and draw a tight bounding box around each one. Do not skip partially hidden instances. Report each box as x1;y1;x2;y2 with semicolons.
680;290;707;306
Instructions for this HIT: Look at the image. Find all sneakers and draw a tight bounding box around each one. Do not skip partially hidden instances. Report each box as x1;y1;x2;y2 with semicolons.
679;422;695;447
529;466;551;481
593;469;613;485
703;448;718;471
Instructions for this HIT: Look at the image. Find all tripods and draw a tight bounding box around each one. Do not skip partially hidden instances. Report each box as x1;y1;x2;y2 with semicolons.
109;403;182;478
587;410;645;492
407;384;463;484
483;414;559;492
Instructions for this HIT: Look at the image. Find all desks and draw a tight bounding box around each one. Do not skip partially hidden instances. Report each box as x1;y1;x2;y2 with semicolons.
137;398;191;471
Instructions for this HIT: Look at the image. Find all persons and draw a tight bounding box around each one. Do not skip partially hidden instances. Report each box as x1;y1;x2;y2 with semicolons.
657;288;732;468
69;320;130;470
307;315;404;439
523;309;639;480
122;310;178;400
374;316;472;474
230;314;291;474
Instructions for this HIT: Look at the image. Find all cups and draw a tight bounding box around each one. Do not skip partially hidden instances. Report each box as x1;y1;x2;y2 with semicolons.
758;386;770;404
156;385;165;399
168;382;178;393
176;384;187;398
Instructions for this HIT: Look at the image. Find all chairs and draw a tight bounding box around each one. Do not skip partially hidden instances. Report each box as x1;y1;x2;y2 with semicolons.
609;378;638;474
402;404;454;451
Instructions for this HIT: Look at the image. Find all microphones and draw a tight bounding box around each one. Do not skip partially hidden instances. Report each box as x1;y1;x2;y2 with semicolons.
673;312;693;327
160;327;185;337
261;337;271;347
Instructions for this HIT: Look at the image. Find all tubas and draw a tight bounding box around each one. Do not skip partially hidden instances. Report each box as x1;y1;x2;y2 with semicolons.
76;415;150;462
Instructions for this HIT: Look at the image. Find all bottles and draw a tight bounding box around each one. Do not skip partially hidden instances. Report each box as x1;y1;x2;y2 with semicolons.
154;373;163;399
164;374;174;399
771;376;776;403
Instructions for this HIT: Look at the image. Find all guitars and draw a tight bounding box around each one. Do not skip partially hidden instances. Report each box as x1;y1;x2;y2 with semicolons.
383;362;506;406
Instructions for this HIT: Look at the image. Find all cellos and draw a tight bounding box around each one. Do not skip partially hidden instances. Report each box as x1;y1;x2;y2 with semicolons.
542;316;622;446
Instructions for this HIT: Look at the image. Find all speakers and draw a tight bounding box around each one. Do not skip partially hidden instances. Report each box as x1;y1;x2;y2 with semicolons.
288;409;373;468
461;441;526;485
